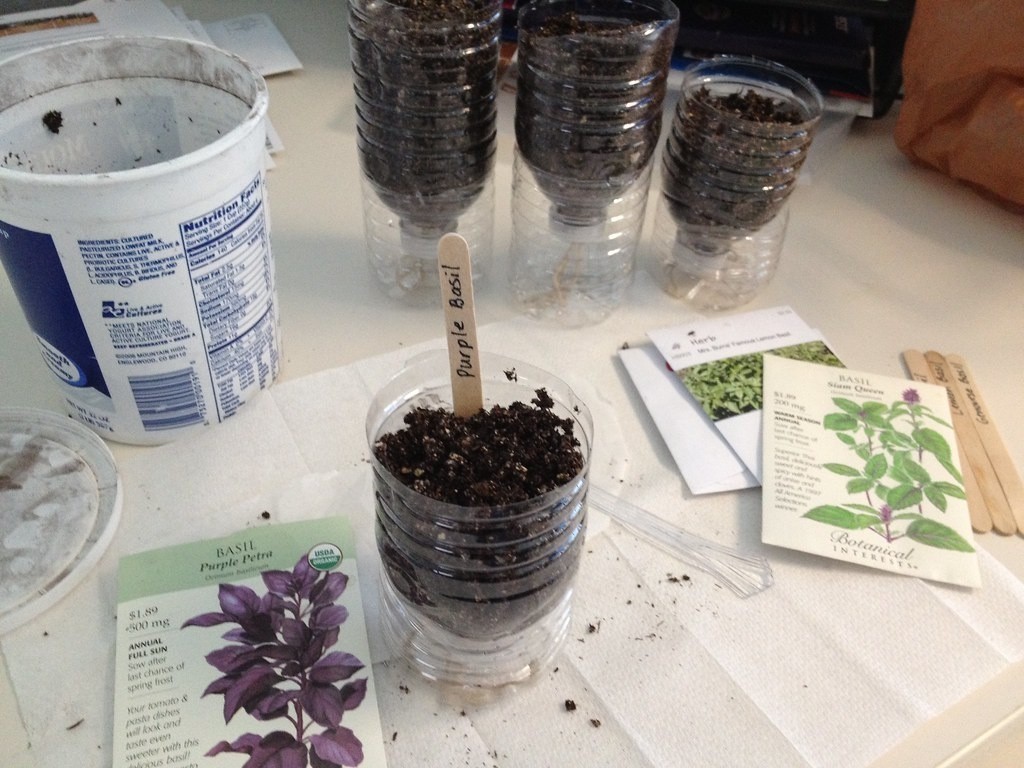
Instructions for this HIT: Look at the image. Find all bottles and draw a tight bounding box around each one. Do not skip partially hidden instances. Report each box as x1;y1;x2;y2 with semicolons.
357;354;592;712
512;4;678;318
652;56;823;311
343;0;507;305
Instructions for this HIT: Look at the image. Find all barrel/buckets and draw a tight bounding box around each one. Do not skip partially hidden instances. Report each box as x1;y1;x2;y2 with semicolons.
0;35;279;444
